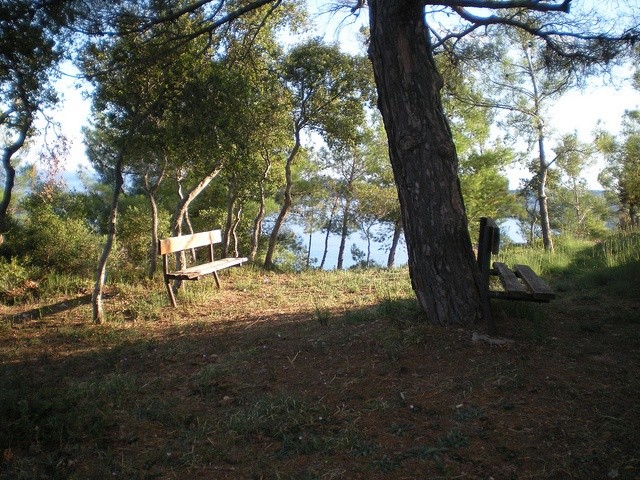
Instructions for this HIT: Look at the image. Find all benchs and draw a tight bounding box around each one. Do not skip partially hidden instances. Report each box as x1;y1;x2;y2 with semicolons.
158;229;249;308
477;216;555;303
